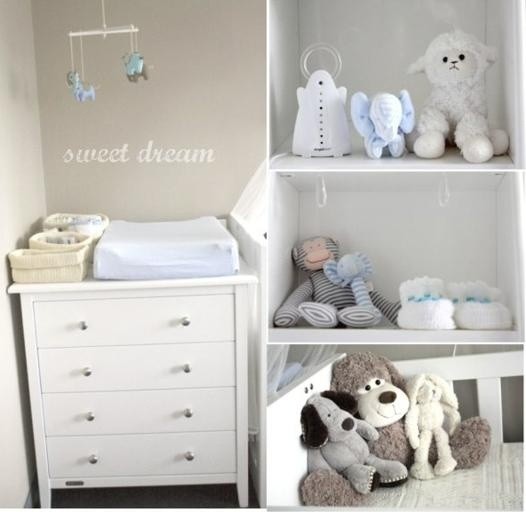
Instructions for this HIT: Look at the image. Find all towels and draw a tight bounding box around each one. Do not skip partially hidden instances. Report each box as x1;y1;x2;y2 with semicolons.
92;215;240;281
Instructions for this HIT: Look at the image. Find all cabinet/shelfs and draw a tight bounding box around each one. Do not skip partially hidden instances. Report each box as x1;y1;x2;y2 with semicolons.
6;252;258;509
266;150;525;348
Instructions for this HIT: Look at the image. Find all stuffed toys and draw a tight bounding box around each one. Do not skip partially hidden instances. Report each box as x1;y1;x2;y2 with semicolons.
123;53;148;84
406;30;510;164
299;349;493;506
299;389;408;494
350;90;416;161
64;70;99;104
274;235;403;327
402;370;461;481
322;249;377;306
